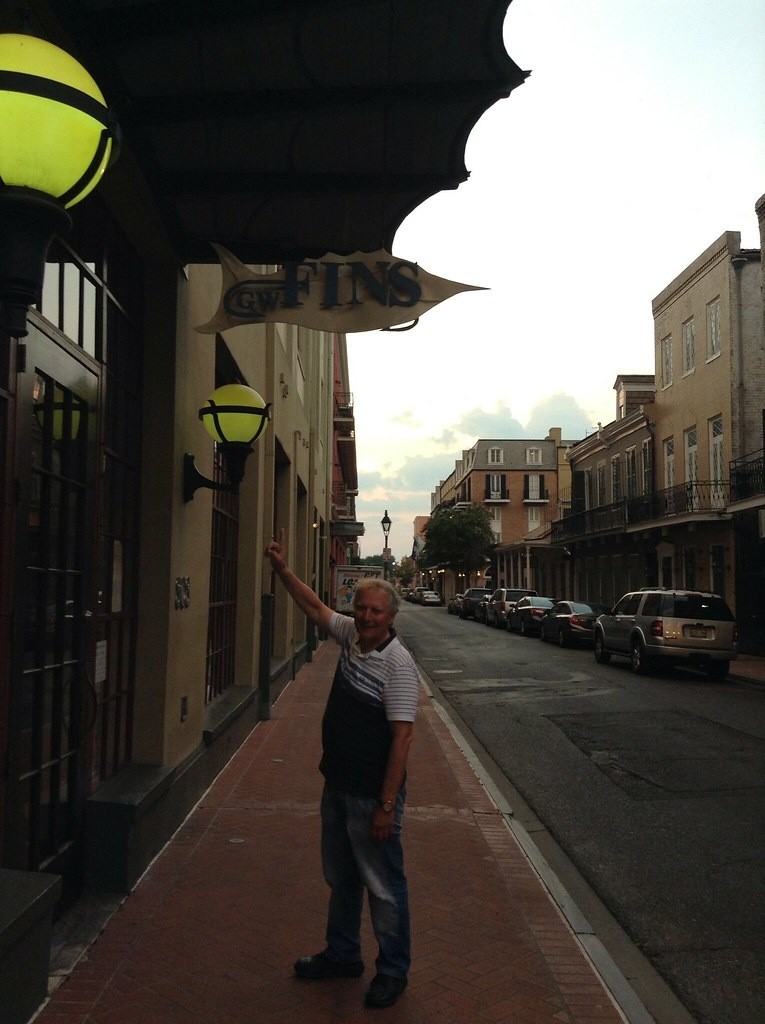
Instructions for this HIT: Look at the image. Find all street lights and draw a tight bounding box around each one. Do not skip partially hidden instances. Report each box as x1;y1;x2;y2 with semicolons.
380;509;390;582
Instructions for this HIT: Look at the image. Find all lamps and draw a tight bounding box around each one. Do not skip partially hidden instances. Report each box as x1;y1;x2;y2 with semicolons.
186;386;277;505
1;30;115;340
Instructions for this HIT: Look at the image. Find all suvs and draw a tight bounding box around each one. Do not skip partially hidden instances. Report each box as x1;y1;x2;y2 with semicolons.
595;586;736;683
458;587;494;621
483;588;538;629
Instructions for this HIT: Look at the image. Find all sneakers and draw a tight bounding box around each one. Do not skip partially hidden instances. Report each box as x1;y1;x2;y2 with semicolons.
364;970;406;1006
295;950;363;978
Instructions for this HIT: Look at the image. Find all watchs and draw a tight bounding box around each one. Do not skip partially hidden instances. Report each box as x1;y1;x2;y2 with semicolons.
377;799;397;813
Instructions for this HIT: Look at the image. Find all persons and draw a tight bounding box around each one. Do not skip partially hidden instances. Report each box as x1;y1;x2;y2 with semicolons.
265;528;418;1009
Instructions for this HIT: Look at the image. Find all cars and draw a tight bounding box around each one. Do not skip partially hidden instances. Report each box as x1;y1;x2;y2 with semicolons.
448;594;464;616
507;596;558;635
476;594;492;622
541;600;613;648
400;585;432;602
422;590;442;605
